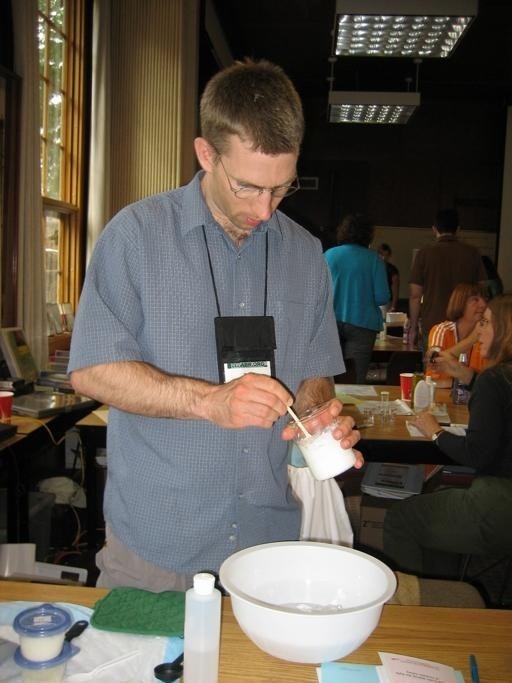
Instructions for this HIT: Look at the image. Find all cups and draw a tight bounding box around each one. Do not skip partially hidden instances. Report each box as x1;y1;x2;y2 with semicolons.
0;391;14;425
290;402;357;482
398;371;414;401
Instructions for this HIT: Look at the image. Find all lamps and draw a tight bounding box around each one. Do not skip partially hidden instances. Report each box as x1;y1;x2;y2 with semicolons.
332;0;478;58
326;57;420;125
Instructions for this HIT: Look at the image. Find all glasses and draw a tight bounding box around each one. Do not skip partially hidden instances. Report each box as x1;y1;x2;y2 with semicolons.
219;158;301;199
479;319;499;328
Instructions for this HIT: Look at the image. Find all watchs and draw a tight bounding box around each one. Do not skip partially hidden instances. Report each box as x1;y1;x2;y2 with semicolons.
431;429;444;446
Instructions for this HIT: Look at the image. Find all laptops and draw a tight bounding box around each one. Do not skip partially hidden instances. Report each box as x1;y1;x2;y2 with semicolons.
11;391;96;419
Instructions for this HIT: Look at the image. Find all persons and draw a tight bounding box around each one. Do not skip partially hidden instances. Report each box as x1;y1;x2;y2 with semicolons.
408;210;481;373
321;213;390;384
424;283;491;392
376;243;399;312
382;295;512;579
67;55;364;593
480;255;503;299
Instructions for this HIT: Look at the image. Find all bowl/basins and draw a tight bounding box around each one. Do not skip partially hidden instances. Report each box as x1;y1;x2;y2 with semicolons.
216;537;399;665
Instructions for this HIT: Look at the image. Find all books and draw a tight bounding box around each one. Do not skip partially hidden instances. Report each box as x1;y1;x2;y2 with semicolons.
361;462;477;500
406;421;467;439
0;327;72;392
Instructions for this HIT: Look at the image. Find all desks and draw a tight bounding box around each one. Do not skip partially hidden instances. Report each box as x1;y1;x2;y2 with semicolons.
369;335;426;363
75;384;485;557
0;415;61;543
0;582;512;683
